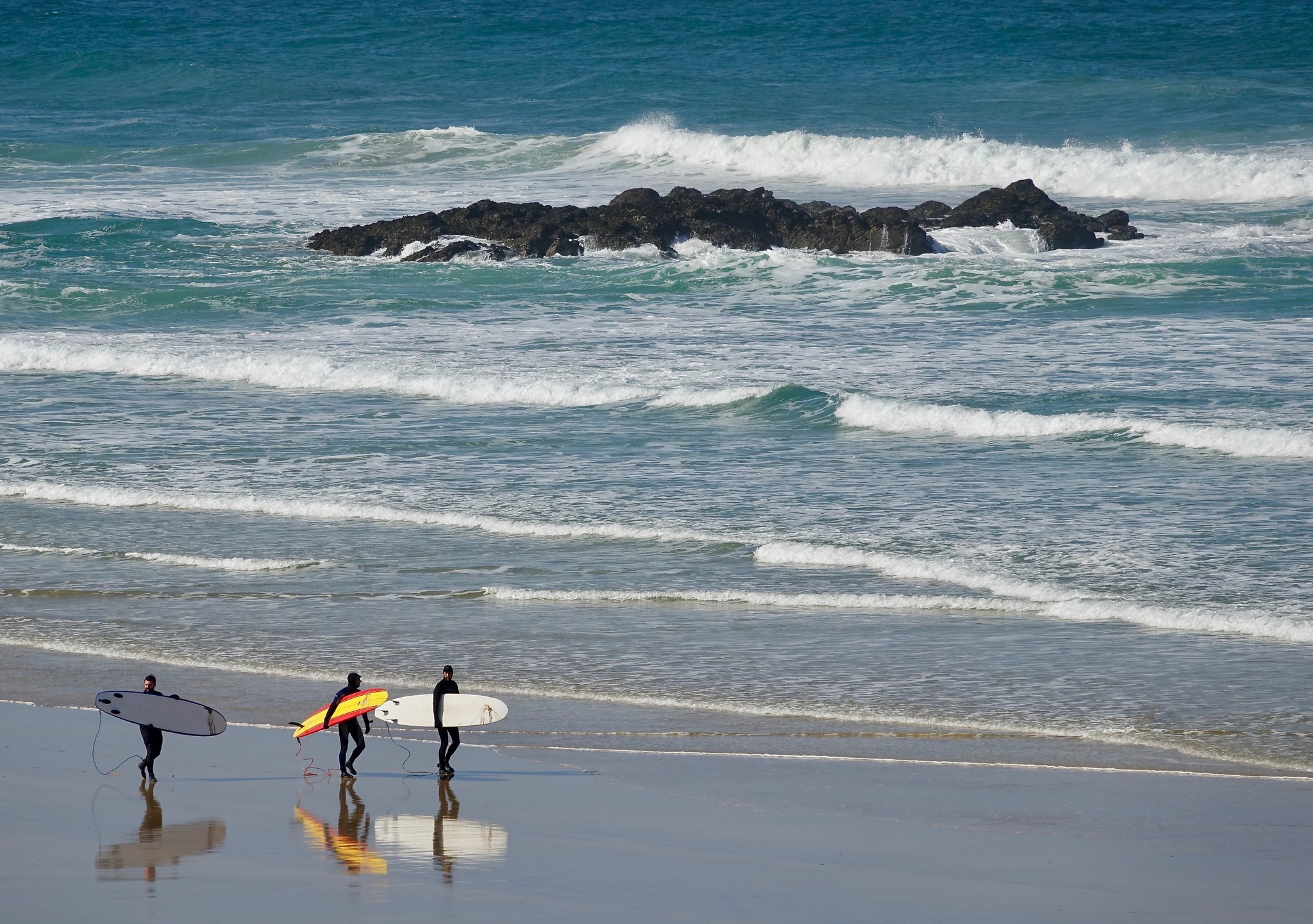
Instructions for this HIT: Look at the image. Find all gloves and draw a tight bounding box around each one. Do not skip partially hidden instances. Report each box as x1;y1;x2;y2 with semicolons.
142;724;153;732
434;720;442;728
169;694;180;700
323;717;329;729
365;724;370;734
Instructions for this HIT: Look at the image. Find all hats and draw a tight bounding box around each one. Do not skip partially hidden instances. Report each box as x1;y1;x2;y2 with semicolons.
443;665;453;680
347;673;361;691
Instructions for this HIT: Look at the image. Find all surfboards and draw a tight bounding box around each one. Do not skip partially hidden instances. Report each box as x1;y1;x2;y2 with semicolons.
94;819;227;871
95;691;227;737
375;814;509;859
288;688;389;738
374;693;508;727
293;805;388;874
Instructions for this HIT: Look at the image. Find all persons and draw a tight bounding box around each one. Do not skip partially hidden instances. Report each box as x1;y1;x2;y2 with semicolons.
324;673;370;778
138;674;179;781
433;665;460;774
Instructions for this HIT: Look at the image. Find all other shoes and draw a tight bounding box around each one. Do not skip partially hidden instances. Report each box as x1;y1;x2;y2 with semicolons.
439;767;452;774
138;762;146;779
345;761;357;775
341;771;353;778
444;757;454;772
149;773;157;781
